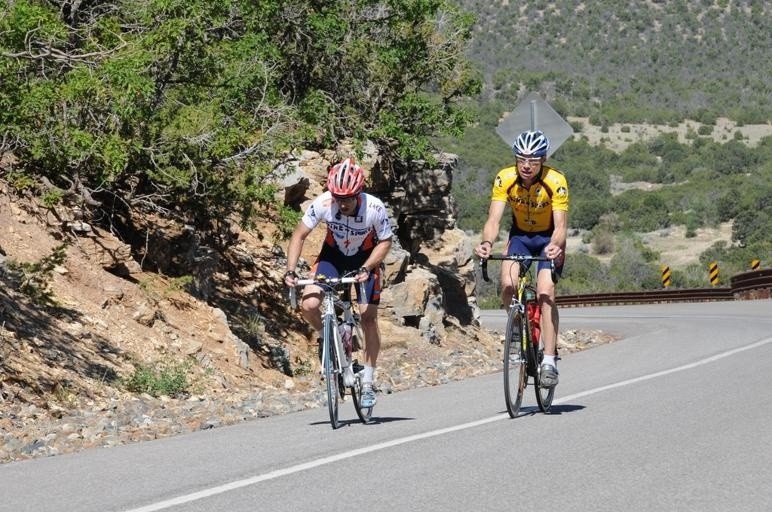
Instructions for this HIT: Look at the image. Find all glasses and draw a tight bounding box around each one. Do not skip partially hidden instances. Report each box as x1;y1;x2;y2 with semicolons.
515;154;541;165
335;198;356;203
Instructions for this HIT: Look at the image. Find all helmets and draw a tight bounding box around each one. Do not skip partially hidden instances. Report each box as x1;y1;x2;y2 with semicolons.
326;157;366;195
513;129;550;159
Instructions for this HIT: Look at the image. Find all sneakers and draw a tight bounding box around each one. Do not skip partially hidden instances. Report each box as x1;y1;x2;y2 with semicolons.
316;338;336;368
360;382;377;407
539;363;559;389
510;332;523;355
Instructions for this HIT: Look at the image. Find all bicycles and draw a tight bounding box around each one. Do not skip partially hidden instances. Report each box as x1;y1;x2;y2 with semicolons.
482;254;561;419
290;270;373;429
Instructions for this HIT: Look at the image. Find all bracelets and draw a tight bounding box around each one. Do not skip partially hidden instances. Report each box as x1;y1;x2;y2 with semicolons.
480;240;493;248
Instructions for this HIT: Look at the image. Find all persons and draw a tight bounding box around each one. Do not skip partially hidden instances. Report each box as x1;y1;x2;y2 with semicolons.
284;158;394;408
473;130;569;388
